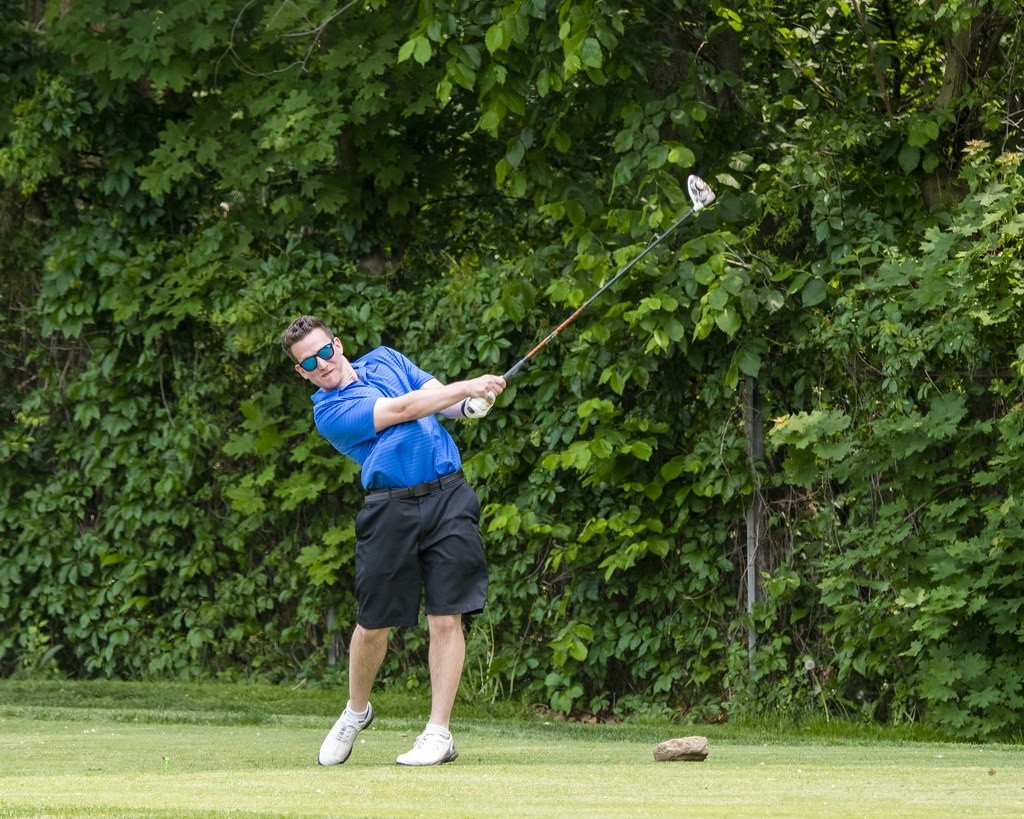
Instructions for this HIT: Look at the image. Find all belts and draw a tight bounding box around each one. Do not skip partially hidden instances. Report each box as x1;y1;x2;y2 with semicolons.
365;469;464;505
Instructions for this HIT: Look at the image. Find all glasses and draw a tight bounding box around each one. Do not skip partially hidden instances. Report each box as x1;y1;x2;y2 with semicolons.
299;340;335;371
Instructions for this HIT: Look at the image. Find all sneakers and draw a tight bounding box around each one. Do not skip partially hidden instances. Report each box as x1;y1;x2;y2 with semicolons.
396;723;459;766
318;701;375;766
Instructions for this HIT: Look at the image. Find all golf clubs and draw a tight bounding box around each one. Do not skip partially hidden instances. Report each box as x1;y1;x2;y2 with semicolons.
466;174;717;415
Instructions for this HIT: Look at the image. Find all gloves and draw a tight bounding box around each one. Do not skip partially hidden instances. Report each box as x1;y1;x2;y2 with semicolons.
461;391;495;419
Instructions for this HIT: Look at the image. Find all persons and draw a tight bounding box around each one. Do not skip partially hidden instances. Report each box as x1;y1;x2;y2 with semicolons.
281;315;506;766
